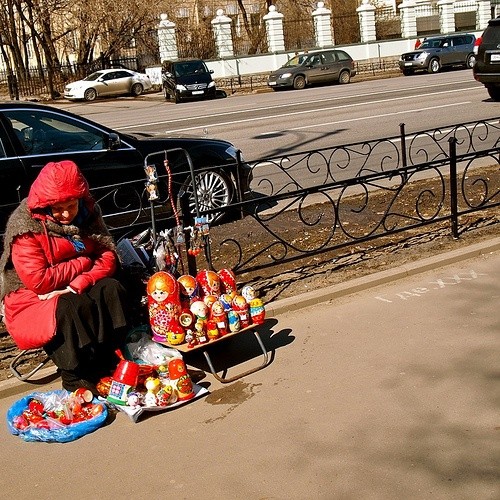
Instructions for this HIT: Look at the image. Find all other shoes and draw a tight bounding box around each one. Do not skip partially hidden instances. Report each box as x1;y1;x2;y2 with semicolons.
61;378;99;397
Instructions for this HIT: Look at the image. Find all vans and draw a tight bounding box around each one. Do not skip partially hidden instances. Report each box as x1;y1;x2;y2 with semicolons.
162;58;217;102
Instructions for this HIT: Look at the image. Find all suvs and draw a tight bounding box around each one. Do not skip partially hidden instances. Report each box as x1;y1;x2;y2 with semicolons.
473;17;500;103
399;33;478;75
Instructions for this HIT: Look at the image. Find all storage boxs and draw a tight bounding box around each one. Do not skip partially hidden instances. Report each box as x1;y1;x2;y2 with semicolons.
117;239;146;269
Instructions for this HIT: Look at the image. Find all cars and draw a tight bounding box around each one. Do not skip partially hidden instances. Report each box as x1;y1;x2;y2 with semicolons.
266;49;356;92
0;101;253;262
62;68;151;101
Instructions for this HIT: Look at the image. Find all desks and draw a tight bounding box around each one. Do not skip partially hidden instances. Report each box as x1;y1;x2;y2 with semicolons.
152;318;270;383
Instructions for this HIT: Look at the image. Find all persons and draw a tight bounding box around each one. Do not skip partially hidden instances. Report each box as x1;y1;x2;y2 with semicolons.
0;161;128;399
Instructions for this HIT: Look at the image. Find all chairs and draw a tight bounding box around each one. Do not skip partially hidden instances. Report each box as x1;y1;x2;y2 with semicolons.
434;42;440;48
